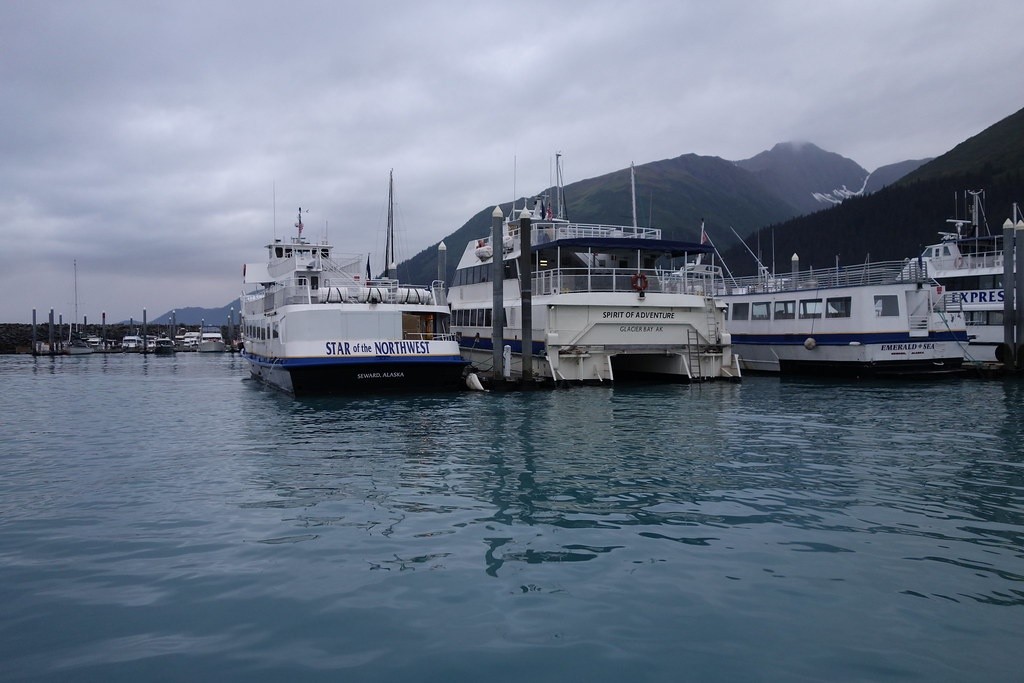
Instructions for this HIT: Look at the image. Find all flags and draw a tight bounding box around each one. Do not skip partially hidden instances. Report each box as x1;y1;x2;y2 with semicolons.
366;256;371;280
547;203;552;221
542;202;546;219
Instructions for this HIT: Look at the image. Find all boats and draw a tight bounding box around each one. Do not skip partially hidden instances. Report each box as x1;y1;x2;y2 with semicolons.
895;187;1024;370
446;149;745;388
653;216;982;383
36;328;201;356
197;318;225;352
239;165;473;400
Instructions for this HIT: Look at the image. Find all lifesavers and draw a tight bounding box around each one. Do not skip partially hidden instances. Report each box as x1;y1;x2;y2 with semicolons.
954;255;964;270
632;274;648;290
